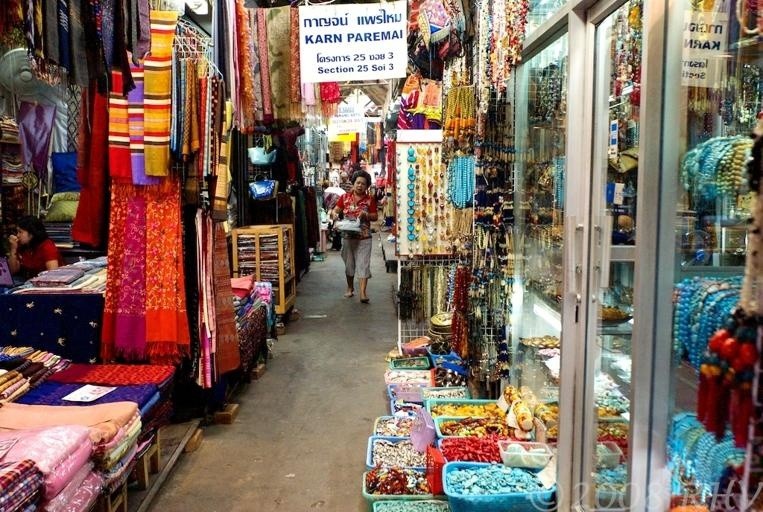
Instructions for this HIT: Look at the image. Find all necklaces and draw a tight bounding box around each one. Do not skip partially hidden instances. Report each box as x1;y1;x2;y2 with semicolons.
396;143;476;260
443;0;531;143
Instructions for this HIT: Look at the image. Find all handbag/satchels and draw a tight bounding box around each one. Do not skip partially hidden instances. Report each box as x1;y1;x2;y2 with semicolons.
248;174;276;198
247;140;277;165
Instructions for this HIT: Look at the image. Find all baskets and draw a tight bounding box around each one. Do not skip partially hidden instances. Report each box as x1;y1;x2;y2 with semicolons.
365;358;555;512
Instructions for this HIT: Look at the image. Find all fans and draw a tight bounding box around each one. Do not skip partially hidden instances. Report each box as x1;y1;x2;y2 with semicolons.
0;47;56;97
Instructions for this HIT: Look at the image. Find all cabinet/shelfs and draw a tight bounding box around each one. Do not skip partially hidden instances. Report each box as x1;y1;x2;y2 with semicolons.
232;222;297;316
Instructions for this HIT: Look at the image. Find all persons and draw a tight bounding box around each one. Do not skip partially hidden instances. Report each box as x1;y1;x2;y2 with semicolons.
322;169;378;302
7;215;66;275
